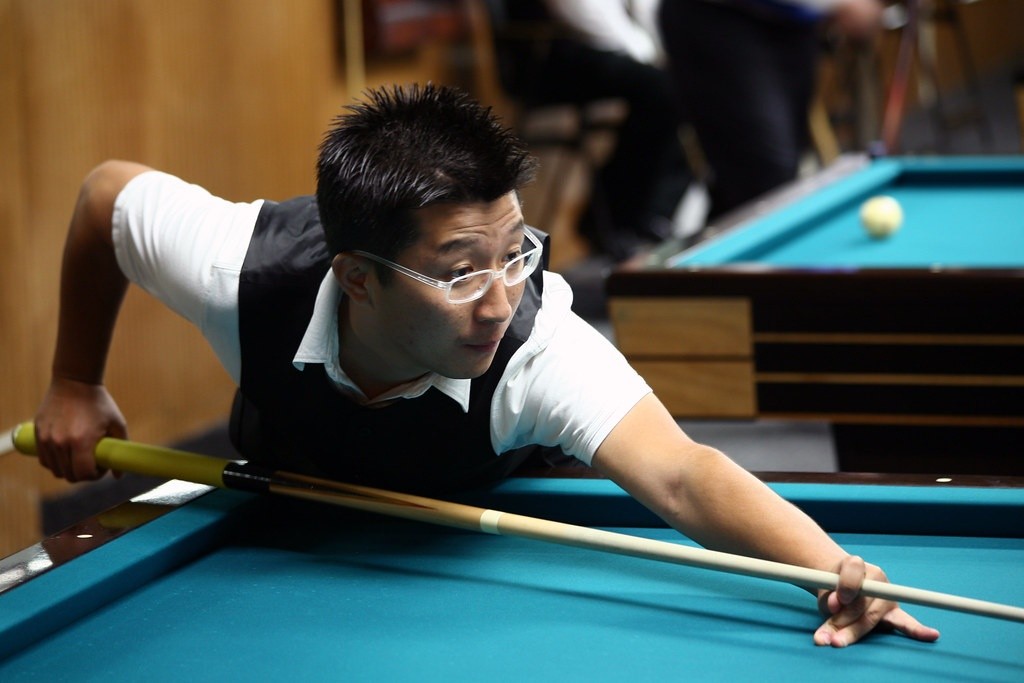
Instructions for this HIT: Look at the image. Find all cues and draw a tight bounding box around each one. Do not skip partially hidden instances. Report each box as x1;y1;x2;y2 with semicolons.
12;420;1023;622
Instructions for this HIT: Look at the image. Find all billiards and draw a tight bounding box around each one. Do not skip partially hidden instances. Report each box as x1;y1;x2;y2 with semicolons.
860;196;902;236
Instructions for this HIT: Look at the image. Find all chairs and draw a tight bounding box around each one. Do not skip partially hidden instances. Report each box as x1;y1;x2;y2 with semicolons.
420;1;626;235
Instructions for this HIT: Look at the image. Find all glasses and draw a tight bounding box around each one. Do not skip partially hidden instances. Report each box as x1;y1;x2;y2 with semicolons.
349;227;543;304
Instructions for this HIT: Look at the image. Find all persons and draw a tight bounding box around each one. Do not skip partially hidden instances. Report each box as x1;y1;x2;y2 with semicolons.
505;0;887;256
34;80;940;647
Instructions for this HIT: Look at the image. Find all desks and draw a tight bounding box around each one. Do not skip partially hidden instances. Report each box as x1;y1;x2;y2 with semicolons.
0;459;1024;683
602;142;1024;474
